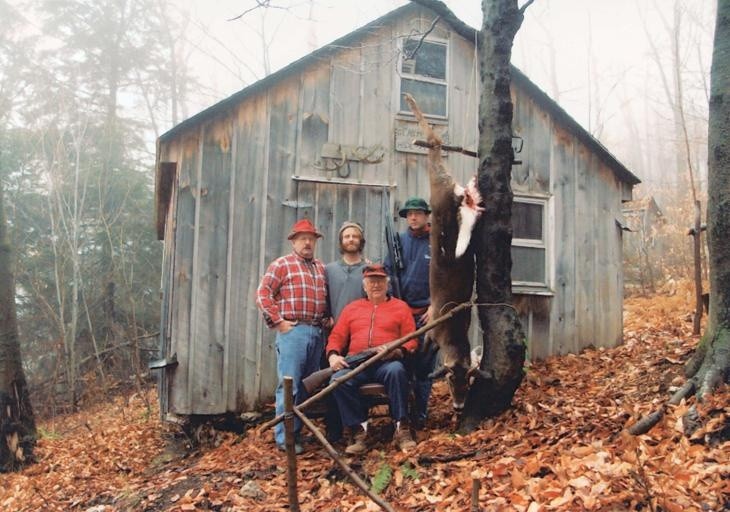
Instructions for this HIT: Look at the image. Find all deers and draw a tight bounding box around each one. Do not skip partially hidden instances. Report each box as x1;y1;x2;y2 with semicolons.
402;90;493;412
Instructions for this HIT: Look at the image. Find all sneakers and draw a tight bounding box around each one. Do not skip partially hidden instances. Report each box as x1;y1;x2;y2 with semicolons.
276;406;465;455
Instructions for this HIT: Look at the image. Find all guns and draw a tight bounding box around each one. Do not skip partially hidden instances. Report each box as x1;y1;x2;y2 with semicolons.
302;337;398;394
383;185;404;299
322;284;342;442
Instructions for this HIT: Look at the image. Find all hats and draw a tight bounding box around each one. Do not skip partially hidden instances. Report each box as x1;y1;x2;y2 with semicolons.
336;220;365;240
399;195;432;218
288;219;323;240
362;263;388;277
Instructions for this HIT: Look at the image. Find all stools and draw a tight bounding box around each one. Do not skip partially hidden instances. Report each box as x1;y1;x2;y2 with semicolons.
355;350;421;430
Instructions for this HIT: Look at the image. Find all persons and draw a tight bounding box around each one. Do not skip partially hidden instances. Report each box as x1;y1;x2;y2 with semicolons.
323;262;422;458
382;199;438;424
321;220;374;446
252;220;330;458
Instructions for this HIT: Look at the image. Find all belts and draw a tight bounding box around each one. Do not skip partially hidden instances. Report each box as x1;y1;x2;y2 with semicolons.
289;317;322;327
411;307;428;315
